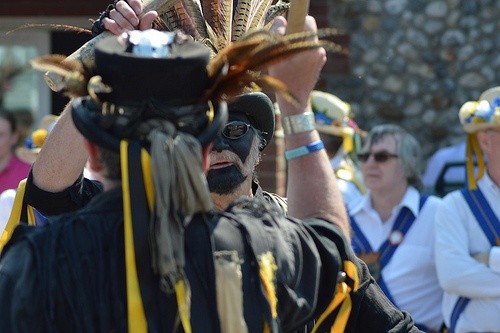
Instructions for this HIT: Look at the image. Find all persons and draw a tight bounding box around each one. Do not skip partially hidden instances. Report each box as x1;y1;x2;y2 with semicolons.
21;1;429;332
344;124;449;332
0;14;364;333
421;132;490;201
430;85;500;332
0;110;32;196
306;90;364;223
14;109;35;149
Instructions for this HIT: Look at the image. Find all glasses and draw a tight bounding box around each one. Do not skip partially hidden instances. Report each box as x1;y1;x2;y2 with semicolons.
356;149;398;163
217;121;251;140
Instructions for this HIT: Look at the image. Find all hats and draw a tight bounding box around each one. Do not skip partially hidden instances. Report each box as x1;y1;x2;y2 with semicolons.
72;30;210;155
309;89;354;137
226;87;275;153
458;87;500;132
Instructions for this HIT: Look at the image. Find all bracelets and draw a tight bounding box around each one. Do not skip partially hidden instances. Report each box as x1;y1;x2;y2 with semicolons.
282;111;317;135
284;139;325;162
